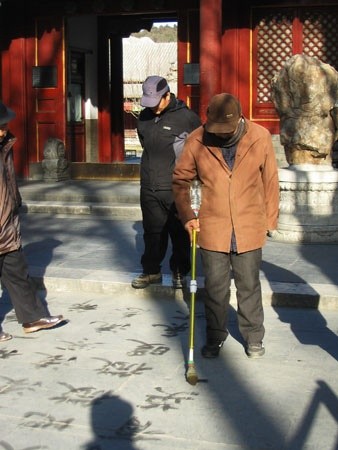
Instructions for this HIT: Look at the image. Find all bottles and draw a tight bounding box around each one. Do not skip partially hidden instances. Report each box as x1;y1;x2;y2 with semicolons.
189;180;202;217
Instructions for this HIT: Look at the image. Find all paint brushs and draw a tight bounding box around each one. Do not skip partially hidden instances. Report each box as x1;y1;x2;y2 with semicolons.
184;226;198;387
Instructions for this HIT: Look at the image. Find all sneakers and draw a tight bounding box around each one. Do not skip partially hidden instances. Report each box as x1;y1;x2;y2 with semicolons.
202;340;224;358
246;341;265;358
132;271;162;289
172;274;187;287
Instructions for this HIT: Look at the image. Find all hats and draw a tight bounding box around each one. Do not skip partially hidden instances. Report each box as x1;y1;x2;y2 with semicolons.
140;75;170;107
205;93;243;134
0;103;16;125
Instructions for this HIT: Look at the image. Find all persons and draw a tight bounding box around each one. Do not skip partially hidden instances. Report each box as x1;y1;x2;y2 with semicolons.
0;102;63;341
131;75;202;289
172;93;279;359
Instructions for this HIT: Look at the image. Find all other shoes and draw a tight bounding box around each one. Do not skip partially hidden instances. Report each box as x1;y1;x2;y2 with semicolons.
0;331;12;341
23;315;64;333
